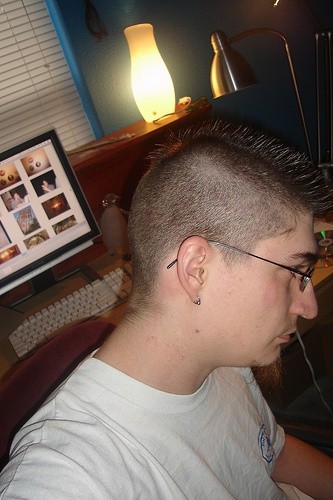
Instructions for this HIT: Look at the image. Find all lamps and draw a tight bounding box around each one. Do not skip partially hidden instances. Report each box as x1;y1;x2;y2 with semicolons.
123;23;176;123
210;28;313;173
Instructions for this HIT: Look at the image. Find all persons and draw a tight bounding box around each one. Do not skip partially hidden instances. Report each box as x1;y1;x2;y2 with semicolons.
11;193;24;210
0;123;333;500
41;180;55;194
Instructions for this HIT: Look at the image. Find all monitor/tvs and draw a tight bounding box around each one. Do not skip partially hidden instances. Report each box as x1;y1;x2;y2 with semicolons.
0;129;103;308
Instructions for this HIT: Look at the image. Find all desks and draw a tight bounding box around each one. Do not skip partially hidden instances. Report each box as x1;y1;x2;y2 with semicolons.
0;210;333;381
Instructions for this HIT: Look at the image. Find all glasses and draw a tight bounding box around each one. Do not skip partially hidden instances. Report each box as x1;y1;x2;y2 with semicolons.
166;237;316;292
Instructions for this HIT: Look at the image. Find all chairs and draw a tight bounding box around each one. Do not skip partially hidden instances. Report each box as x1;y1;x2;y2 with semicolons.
1;317;333;500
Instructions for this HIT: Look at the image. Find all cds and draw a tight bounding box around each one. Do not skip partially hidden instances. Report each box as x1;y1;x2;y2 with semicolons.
314;230;333;259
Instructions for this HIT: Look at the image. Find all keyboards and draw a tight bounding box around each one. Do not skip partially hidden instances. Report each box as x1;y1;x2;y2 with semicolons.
8;261;133;361
